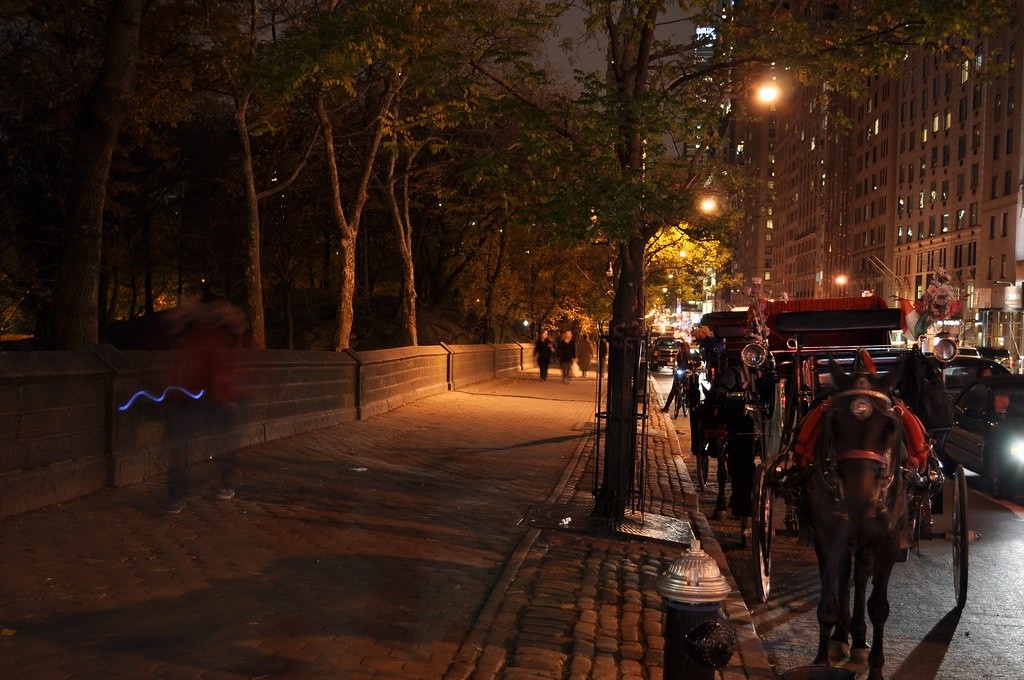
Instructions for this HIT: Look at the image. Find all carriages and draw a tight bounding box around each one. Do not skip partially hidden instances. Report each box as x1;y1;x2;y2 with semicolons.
687;310;786;532
739;291;982;680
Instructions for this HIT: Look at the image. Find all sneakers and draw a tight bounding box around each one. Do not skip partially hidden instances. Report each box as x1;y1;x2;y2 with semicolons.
165;502;187;515
217;487;234;499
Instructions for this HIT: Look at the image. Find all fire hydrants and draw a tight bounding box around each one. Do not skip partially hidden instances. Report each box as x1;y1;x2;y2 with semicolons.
654;537;738;680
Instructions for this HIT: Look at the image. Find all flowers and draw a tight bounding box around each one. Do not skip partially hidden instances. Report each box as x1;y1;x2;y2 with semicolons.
913;267;962;340
692;325;713;347
744;276;771;344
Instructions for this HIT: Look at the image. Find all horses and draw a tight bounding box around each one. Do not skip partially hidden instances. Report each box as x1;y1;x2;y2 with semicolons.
792;357;943;680
701;366;754;522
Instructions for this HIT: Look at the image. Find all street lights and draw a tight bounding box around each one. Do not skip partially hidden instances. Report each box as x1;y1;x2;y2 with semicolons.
752;72;851;298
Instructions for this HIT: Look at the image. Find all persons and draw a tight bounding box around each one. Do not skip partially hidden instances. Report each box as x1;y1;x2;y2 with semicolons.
595;335;607;378
660;342;691;413
532;329;576;382
978;367;992;376
149;273;247;516
994;393;1010;414
576;333;593;377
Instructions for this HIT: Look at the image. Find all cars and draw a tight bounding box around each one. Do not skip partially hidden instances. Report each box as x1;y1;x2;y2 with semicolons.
934;374;1024;501
922;342;1024;407
647;326;706;373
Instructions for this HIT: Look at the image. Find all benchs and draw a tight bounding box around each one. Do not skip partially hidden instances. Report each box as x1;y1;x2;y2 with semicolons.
771;307;901;383
716;325;746;360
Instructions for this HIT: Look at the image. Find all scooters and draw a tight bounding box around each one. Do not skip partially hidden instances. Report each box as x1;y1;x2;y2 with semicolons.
670;366;690;420
